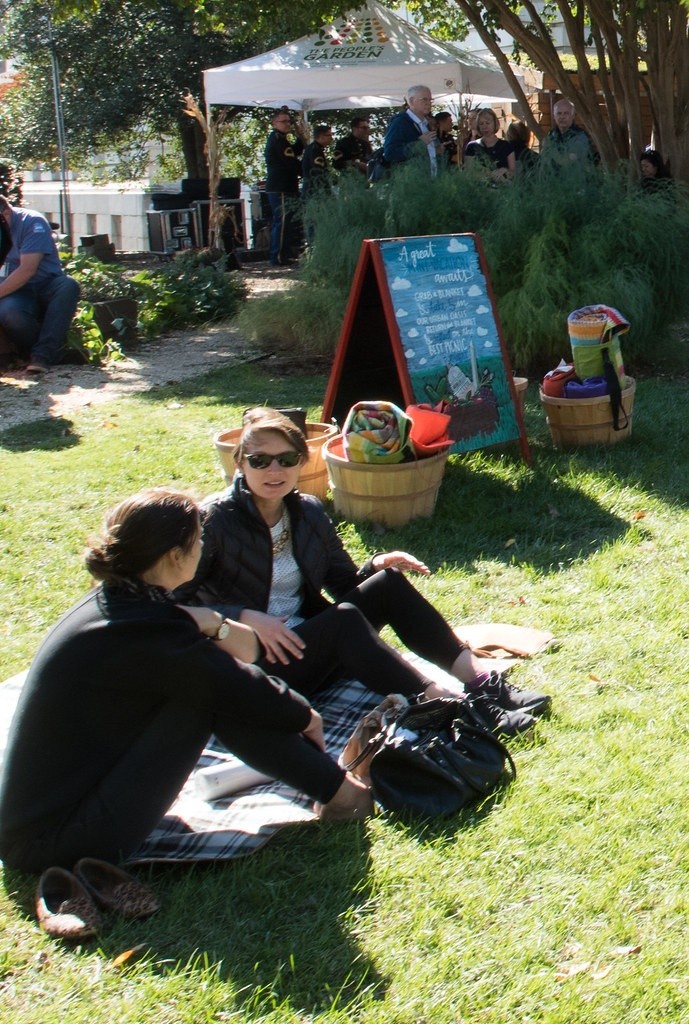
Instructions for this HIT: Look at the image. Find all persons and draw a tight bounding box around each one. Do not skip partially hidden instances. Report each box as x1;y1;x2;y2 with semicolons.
0;195;79;374
266;84;673;274
169;407;551;741
0;488;377;876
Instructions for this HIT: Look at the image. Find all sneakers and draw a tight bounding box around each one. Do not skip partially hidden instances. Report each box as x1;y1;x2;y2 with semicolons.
25;354;49;374
71;348;90;364
464;692;534;742
471;670;552;715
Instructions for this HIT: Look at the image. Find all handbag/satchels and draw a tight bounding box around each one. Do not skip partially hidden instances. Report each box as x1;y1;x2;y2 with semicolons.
366;696;516;826
336;693;409;789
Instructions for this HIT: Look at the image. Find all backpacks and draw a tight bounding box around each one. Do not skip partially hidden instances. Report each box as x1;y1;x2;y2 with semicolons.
366;146;390;184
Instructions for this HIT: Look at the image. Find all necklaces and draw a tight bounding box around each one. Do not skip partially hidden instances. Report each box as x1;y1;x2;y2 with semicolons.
273;502;288;555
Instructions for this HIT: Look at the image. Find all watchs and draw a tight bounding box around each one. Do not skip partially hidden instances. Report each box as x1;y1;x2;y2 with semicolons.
205;613;230;641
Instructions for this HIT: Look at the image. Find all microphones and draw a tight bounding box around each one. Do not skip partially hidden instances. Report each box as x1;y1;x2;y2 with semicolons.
453;125;458;130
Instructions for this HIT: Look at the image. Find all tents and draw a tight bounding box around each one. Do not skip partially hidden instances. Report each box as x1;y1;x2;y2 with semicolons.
202;1;543;176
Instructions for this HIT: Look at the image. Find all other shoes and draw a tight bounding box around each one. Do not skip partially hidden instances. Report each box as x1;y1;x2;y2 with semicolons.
73;857;162;919
35;867;101;938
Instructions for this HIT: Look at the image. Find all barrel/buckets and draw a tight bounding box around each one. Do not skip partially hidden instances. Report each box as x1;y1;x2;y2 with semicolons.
213;421;338;505
321;433;451;528
539;375;638;450
513;377;528;412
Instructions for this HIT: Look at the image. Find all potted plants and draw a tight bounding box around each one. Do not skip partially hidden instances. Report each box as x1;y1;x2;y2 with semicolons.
77;268;138;349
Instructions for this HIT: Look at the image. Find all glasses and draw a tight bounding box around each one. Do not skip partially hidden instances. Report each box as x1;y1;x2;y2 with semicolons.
357;126;370;130
646;149;653;156
241;450;303;470
323;134;332;138
275;120;291;125
413;98;433;103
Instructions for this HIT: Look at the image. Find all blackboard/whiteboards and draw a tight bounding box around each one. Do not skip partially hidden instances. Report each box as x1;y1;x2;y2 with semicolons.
319;232;525;454
